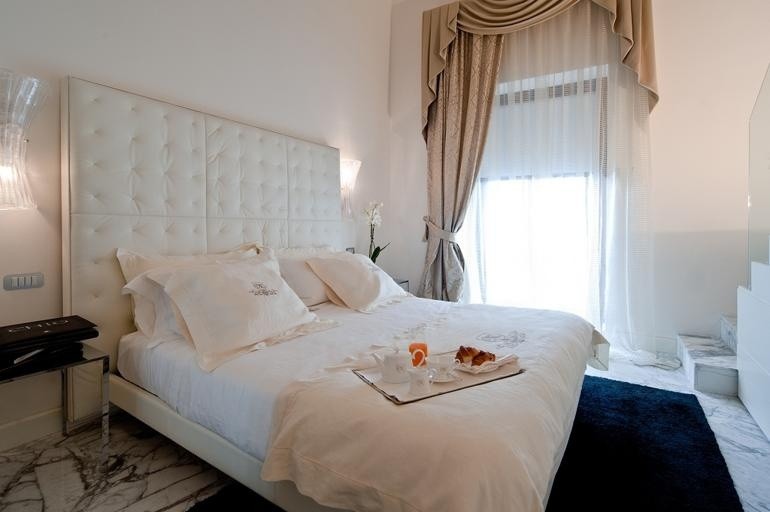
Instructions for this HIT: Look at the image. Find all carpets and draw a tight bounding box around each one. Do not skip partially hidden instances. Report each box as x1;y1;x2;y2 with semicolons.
187;373;745;512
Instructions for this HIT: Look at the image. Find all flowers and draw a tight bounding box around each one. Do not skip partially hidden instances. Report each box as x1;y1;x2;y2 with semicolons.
362;200;392;261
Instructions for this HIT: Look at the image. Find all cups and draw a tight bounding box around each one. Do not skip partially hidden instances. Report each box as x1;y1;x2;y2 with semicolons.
409;343;458;382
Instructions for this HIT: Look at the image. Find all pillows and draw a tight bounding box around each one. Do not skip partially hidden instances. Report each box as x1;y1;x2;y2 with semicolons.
115;245;410;374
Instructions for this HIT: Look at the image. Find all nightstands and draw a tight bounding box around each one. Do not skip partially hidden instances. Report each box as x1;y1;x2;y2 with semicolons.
0;344;111;473
397;280;409;293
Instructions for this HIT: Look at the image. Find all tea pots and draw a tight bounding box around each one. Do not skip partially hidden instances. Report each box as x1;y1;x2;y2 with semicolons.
370;347;424;385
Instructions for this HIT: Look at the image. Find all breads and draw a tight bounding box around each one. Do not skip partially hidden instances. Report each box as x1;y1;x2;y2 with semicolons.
455;345;479;363
471;350;495;367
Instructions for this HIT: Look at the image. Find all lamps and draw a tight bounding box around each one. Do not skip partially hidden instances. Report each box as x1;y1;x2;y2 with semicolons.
0;67;49;213
339;157;362;224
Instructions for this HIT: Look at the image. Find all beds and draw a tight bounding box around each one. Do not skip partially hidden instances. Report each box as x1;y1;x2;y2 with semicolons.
59;71;611;510
